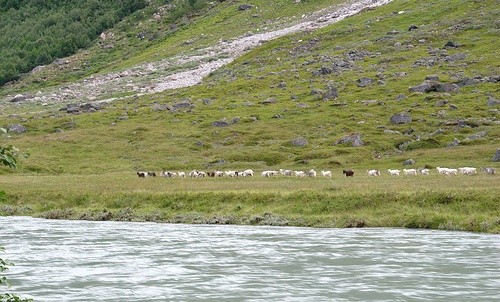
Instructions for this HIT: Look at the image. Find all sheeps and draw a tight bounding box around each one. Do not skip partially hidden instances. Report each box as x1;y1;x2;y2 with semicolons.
137;166;497;178
